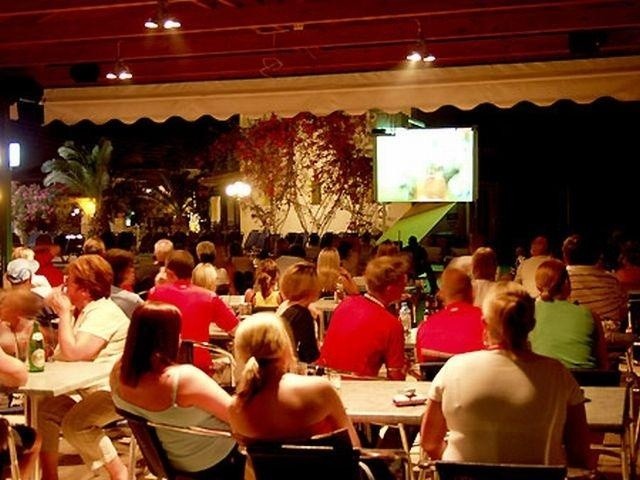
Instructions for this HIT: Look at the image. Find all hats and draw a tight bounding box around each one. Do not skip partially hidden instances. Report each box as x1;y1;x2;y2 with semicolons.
6;257;40;284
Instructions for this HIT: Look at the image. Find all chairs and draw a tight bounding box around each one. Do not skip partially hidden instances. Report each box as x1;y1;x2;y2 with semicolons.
0;230;640;480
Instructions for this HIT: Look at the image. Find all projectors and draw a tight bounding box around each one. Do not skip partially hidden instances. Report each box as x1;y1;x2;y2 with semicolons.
372;128;385;133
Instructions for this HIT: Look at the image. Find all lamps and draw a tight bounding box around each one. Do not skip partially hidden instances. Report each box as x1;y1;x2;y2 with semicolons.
226;181;252;201
406;35;436;62
144;0;181;30
106;58;132;80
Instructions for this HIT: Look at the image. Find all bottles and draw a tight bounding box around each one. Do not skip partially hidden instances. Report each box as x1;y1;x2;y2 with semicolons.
28;320;46;371
398;273;436;335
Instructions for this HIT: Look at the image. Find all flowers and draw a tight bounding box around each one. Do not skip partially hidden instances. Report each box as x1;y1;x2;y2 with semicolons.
10;181;57;244
